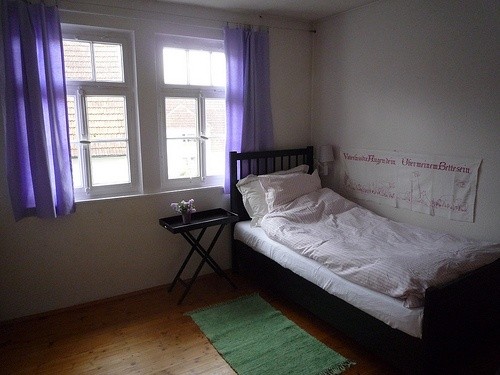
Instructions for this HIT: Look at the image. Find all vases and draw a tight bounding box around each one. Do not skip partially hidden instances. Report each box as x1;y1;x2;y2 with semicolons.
182;214;191;224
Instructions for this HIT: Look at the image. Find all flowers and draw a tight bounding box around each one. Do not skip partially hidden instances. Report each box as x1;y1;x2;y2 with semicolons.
170;199;196;214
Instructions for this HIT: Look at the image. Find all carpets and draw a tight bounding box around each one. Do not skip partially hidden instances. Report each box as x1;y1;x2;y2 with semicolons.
184;290;357;375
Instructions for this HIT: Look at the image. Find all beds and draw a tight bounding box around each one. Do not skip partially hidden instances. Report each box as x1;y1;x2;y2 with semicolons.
230;145;500;375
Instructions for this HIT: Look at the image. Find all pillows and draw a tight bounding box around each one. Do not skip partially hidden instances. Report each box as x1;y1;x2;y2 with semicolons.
258;169;322;212
236;164;310;227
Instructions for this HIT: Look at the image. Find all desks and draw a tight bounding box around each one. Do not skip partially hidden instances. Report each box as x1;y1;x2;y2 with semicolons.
159;208;240;305
